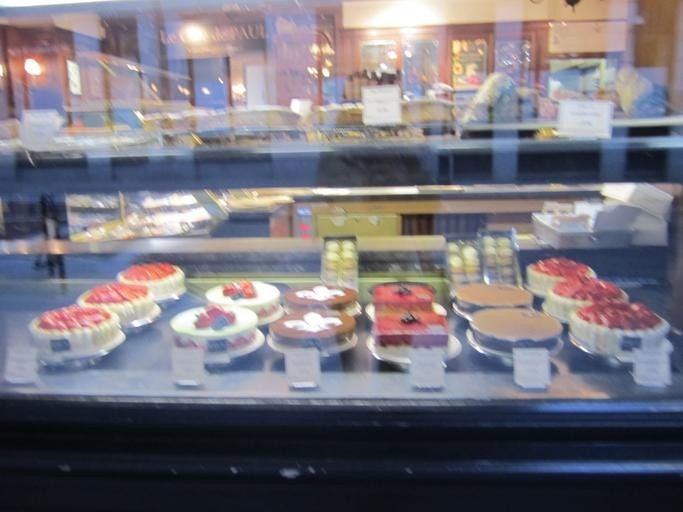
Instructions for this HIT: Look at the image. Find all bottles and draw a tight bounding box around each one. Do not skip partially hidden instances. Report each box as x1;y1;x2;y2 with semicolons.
337;68;395;101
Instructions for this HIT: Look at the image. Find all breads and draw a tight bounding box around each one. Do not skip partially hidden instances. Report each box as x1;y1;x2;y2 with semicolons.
447;234;515;274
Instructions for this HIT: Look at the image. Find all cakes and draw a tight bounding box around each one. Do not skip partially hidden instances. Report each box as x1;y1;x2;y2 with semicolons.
170;303;259;355
268;283;360;352
116;259;186;300
205;278;283;325
372;282;450;357
544;275;628;321
569;297;671;357
526;257;597;294
454;283;560;353
29;303;121;358
76;281;154;325
